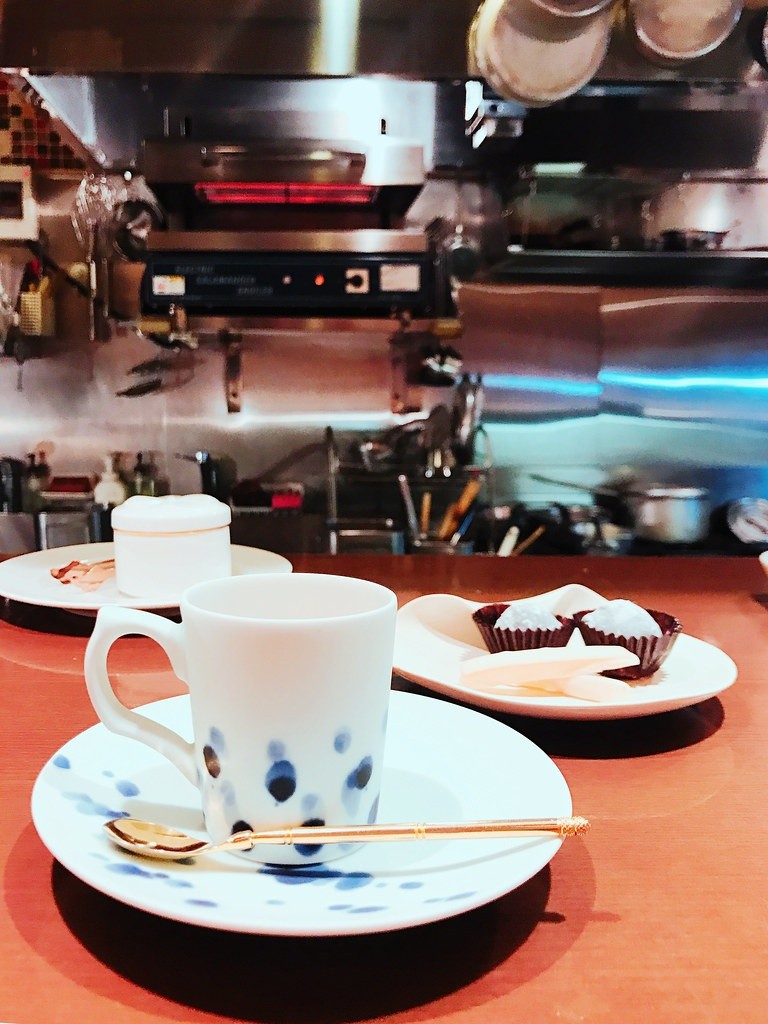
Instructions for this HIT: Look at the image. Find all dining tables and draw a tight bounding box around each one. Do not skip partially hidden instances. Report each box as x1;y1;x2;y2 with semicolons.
0;554;768;1024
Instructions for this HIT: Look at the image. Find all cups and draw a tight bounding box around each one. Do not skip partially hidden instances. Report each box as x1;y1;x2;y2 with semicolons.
203;144;248;183
408;537;476;555
109;503;234;596
86;571;399;864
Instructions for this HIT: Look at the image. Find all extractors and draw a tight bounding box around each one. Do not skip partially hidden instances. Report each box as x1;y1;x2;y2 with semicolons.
142;231;447;322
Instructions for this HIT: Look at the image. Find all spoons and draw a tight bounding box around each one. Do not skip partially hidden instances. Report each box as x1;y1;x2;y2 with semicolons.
108;812;592;864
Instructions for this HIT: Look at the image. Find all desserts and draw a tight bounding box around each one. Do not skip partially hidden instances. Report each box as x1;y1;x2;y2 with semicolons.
473;598;684;680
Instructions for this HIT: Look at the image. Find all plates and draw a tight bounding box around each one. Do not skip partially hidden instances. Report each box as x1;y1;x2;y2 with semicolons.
0;542;293;620
392;628;740;724
31;691;574;940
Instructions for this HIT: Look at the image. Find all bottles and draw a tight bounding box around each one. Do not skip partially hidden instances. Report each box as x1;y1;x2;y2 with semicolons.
19;448;157;516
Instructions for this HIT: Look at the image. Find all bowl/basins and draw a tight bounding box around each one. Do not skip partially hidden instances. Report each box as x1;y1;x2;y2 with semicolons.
259;145;365;186
662;227;729;252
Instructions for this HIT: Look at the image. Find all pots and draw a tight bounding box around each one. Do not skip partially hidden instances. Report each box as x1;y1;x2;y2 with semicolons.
468;0;768;112
514;210;602;249
528;473;713;544
